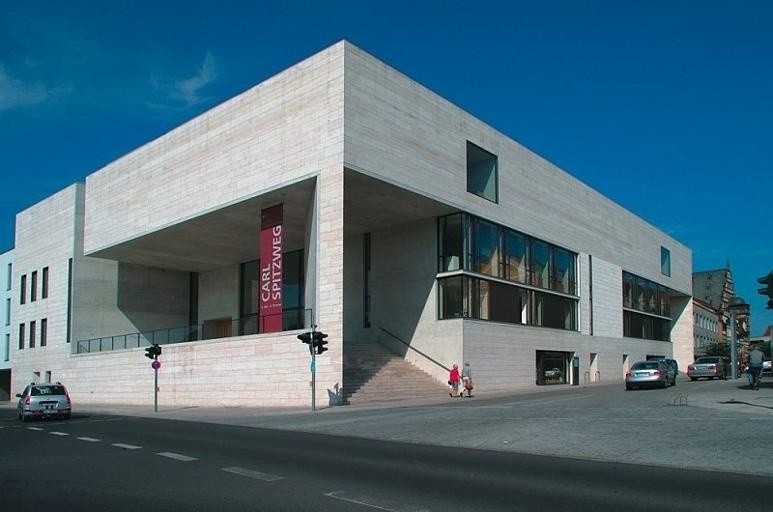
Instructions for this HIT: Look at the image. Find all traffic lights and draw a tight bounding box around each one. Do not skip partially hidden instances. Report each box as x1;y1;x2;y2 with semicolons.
155;346;161;354
297;332;310;344
317;332;328;354
144;346;152;358
756;271;771;298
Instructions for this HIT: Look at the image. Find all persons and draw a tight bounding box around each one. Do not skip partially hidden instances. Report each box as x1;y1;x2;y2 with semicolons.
459;362;476;397
748;345;767;389
448;363;460;398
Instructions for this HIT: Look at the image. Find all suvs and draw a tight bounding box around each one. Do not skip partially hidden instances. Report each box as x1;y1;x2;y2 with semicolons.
15;381;71;421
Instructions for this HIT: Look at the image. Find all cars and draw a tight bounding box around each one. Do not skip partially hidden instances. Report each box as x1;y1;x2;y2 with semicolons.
544;368;562;379
648;357;678;377
686;356;728;381
625;359;676;390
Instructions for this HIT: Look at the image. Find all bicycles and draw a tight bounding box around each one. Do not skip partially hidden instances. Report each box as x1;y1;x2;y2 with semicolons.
751;367;761;390
735;361;748;378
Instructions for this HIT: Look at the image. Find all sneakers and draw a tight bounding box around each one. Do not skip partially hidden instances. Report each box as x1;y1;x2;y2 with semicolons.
449;393;474;397
